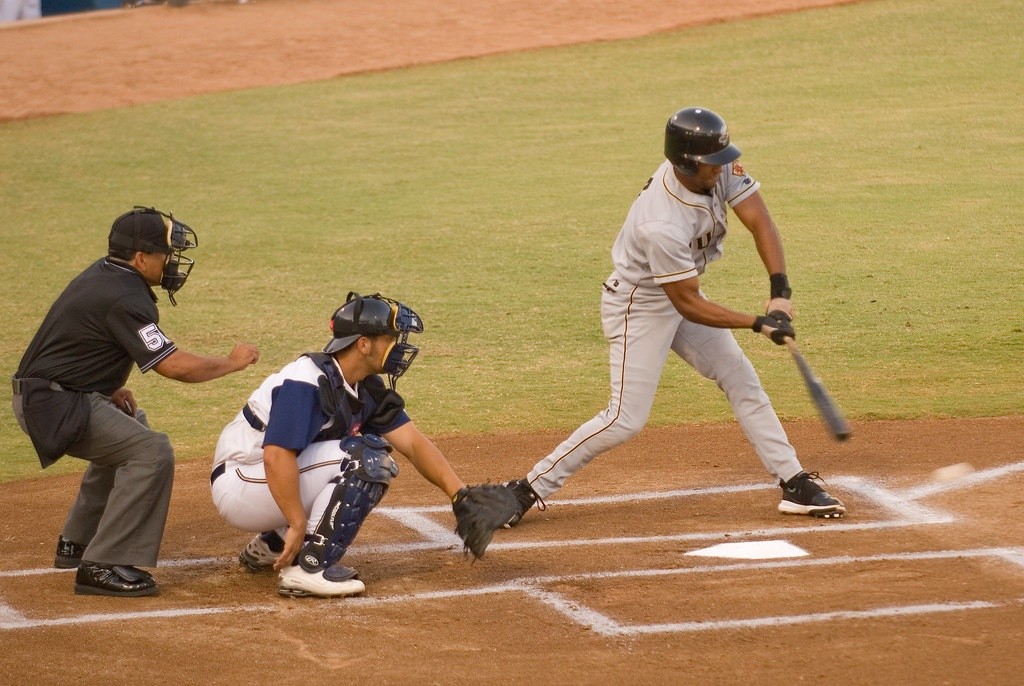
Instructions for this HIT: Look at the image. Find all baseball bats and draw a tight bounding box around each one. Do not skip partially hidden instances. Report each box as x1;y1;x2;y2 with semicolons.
782;335;852;442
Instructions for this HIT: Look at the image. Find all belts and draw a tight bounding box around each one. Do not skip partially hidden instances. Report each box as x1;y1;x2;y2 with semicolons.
12;379;23;394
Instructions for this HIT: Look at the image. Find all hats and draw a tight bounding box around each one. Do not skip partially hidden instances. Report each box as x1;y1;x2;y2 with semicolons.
108;208;192;255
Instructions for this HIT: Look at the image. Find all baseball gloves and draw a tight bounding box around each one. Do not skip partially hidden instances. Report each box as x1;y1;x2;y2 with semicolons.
451;477;518;560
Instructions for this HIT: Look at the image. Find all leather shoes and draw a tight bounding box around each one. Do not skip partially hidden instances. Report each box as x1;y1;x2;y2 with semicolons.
54;535;86;568
74;561;157;597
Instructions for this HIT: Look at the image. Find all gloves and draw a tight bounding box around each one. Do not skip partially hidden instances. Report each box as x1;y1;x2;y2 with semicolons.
754;314;795;346
766;298;794;321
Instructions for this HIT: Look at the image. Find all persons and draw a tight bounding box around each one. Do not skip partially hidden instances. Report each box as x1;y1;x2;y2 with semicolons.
12;205;258;596
502;107;847;529
209;292;518;598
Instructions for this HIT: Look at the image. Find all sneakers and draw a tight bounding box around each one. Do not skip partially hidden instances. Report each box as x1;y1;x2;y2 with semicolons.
500;479;546;530
778;471;846;518
239;532;284;573
277;562;365;598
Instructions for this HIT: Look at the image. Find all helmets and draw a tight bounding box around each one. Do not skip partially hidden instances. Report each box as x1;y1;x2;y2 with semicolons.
664;107;742;177
322;293;394;353
